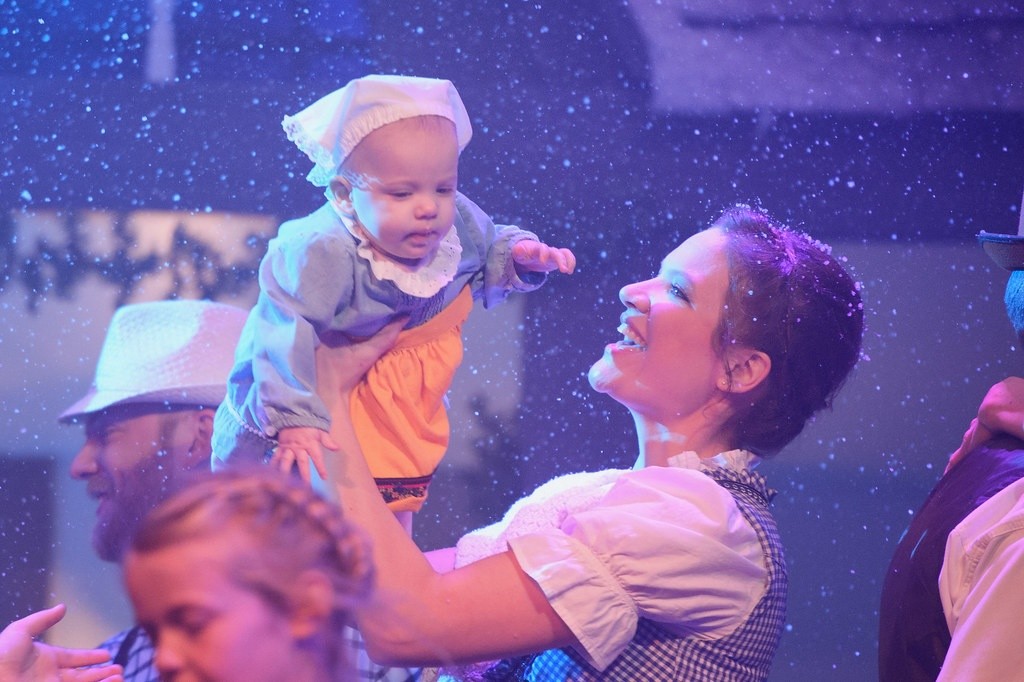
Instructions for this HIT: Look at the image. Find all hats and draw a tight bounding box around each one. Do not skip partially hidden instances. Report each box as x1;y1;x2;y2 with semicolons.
55;301;248;426
279;75;473;194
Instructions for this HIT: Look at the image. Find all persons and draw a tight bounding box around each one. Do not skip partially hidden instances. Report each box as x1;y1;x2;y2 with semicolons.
57;297;384;680
1;605;122;680
290;205;866;682
125;464;375;680
874;192;1023;682
206;72;577;539
